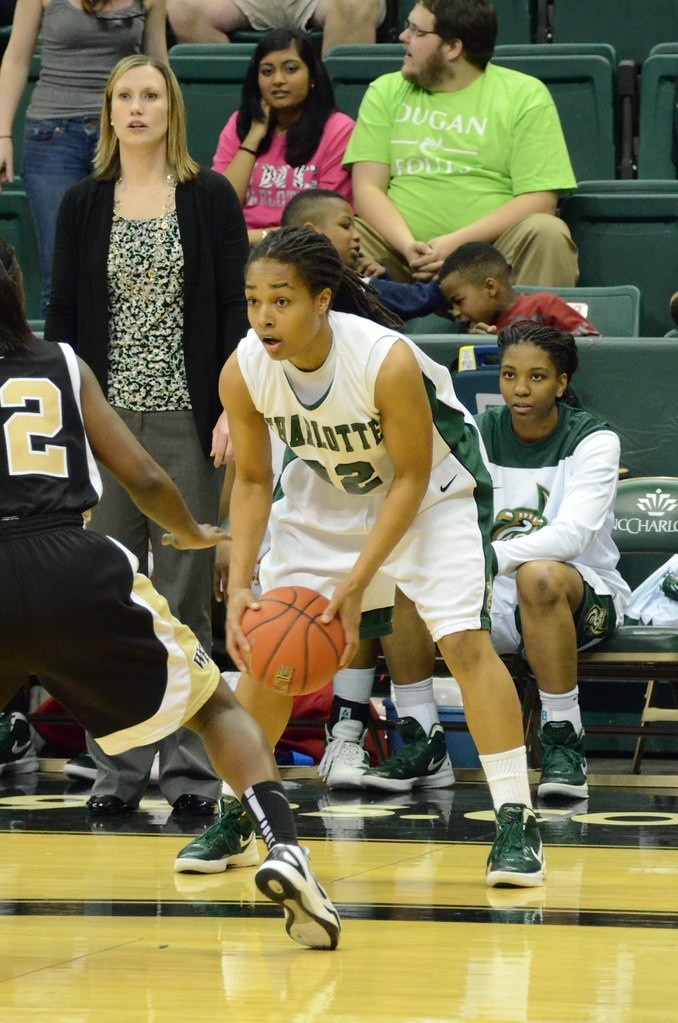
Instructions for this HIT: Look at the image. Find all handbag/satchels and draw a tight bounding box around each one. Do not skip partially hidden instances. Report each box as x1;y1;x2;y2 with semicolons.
274;679;388;767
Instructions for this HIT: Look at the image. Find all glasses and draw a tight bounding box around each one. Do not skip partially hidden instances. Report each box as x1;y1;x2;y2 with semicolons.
95;0;147;20
403;20;435;36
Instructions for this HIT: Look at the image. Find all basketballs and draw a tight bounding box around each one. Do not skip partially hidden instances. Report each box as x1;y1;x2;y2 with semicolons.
236;584;348;699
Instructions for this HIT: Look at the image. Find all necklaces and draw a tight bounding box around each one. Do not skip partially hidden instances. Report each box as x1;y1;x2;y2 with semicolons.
111;169;177;304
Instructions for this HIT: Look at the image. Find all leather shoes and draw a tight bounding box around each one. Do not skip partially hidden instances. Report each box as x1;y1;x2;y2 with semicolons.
175;793;259;874
86;795;128;815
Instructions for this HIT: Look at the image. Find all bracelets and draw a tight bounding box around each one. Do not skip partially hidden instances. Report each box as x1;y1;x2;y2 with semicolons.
239;146;258;157
0;135;12;138
260;228;266;240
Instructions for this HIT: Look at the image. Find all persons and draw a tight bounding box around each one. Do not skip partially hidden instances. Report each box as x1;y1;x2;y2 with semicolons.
166;0;386;62
0;0;166;318
174;227;548;889
45;55;249;824
0;454;237;783
342;0;582;290
437;241;602;336
280;188;452;324
0;237;341;952
361;320;632;800
209;27;357;250
316;639;379;792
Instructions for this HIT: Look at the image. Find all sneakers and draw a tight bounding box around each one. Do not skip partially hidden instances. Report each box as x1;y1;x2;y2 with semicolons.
174;794;221;815
361;717;456;793
318;719;370;790
486;803;547;888
255;844;341;950
538;721;588;799
0;712;40;776
65;750;98;781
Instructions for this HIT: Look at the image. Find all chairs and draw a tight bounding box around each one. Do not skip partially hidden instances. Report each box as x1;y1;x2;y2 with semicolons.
0;0;678;784
